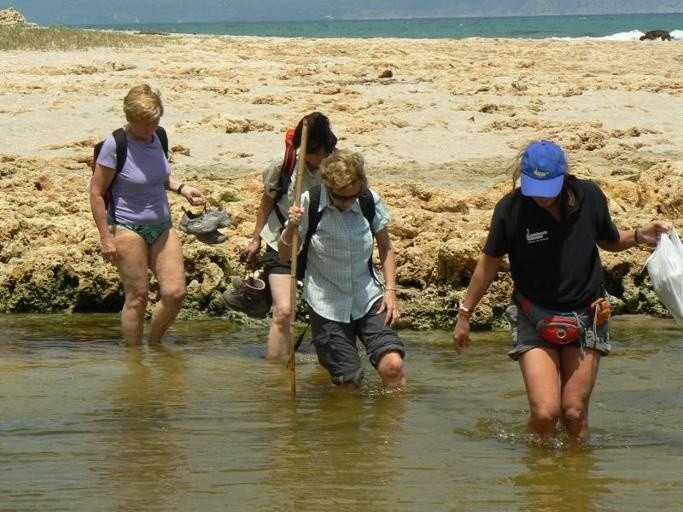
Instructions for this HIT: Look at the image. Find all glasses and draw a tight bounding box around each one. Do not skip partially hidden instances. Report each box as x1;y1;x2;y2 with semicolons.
328;180;363;201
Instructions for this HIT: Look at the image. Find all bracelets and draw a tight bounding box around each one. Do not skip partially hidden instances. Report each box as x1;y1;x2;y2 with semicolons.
384;288;398;292
457;303;475;318
278;228;299;248
634;227;643;246
177;183;185;194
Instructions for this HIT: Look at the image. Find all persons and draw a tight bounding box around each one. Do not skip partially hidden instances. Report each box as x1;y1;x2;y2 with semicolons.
240;112;345;358
89;83;208;347
277;148;408;389
452;139;674;440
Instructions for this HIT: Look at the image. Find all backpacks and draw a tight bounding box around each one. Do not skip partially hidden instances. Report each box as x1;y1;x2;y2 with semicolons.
91;126;167;212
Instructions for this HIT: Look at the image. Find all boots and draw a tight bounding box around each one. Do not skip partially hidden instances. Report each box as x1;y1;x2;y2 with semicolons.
223;277;272;319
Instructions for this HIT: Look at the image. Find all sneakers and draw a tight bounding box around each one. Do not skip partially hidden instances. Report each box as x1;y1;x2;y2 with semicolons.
179;206;233;245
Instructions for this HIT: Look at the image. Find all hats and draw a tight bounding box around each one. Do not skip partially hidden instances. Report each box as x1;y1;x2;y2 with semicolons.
519;139;567;200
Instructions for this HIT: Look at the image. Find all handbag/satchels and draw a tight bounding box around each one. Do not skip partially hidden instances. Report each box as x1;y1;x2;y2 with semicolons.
520;299;589;347
